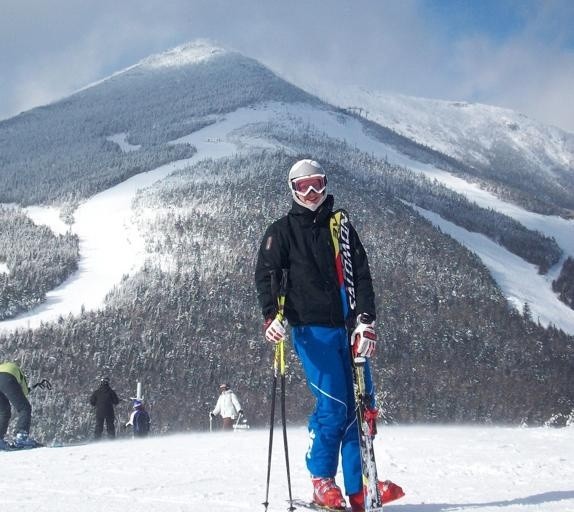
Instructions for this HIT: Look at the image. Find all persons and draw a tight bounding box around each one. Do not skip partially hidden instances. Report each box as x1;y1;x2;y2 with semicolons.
90;376;151;440
0;361;43;451
211;383;244;432
255;158;405;512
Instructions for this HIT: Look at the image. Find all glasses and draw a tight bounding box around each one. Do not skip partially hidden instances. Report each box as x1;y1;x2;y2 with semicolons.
292;174;326;196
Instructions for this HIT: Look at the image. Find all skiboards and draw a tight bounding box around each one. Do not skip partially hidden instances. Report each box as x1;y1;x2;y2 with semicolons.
0;441;49;452
328;208;385;512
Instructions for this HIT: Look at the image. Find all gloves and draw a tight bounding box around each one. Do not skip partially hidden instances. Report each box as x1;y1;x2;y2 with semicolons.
350;313;379;357
262;318;288;343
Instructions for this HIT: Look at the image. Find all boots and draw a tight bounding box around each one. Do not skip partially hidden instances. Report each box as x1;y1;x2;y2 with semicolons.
349;479;405;512
0;432;36;449
311;478;346;510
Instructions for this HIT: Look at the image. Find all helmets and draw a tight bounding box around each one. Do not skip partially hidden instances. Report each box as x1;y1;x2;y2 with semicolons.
288;159;327;191
